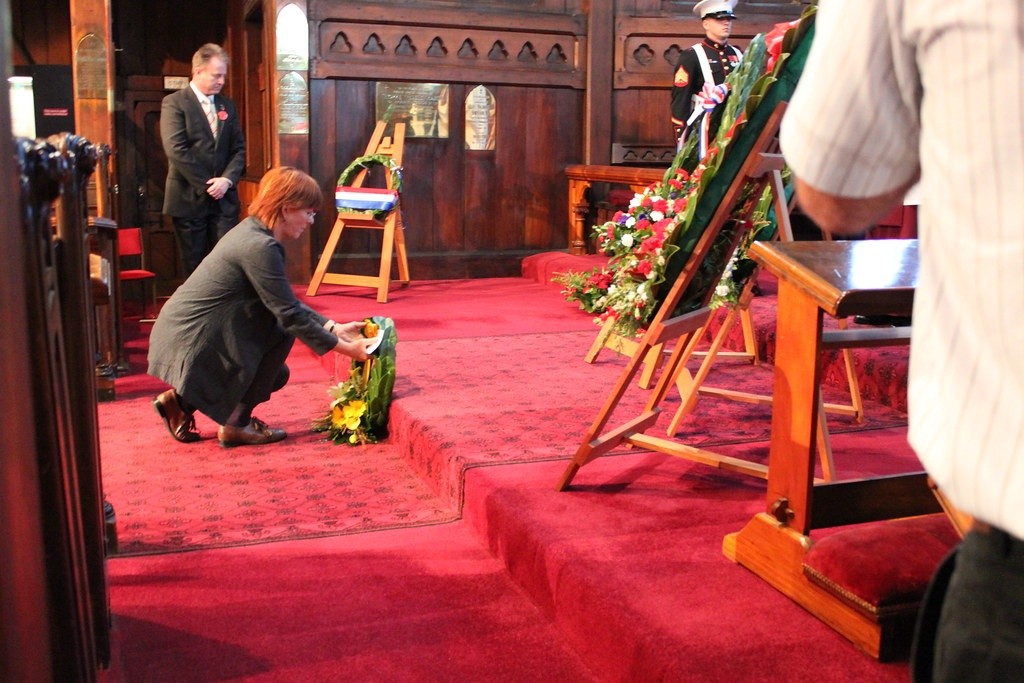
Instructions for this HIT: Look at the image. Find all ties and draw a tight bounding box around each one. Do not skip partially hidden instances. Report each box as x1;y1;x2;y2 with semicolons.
203;98;217;139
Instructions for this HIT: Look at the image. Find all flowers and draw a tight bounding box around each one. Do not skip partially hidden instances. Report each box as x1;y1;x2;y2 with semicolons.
546;131;790;331
313;314;403;452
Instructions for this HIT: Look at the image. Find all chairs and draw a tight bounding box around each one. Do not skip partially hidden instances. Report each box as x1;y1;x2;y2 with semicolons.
116;227;158;319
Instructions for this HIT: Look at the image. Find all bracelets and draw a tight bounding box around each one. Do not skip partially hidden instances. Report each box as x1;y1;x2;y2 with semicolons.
329;321;338;333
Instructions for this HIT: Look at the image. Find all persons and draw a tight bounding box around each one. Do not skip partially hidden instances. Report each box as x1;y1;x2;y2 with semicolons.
147;165;379;448
778;0;1024;683
671;0;745;154
162;43;245;280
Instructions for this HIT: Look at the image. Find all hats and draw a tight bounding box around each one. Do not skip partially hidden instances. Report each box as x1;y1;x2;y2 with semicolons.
693;0;739;19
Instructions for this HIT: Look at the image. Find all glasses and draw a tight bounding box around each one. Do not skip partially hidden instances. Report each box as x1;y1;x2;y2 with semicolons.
302;208;317;219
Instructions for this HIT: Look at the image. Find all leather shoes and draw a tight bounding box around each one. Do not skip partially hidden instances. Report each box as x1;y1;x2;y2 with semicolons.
218;416;287;447
154;388;200;442
